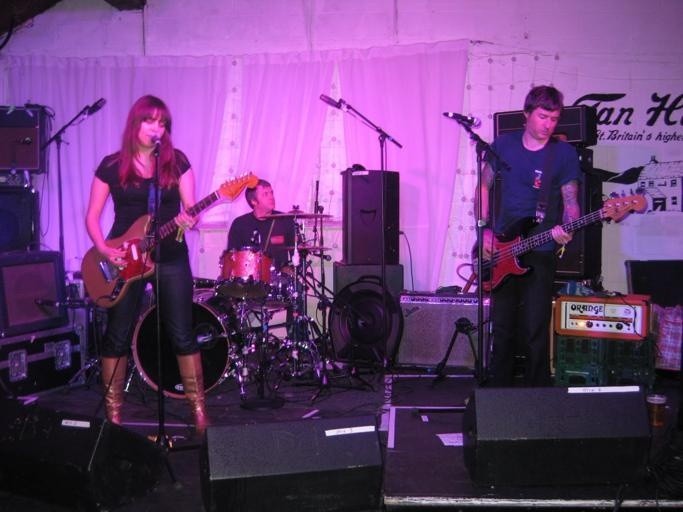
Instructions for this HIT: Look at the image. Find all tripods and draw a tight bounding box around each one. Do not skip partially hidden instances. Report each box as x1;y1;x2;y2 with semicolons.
152;157;202;491
222;217;377;407
410;125;511;419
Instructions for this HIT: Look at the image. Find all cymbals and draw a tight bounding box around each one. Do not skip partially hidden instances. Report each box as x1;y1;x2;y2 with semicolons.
258;211;333;219
272;245;330;252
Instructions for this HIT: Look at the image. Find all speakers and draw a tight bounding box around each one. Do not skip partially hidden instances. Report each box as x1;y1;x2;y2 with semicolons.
462;386;649;486
200;415;383;512
332;260;404;368
340;164;399;265
0;182;39;252
0;399;167;512
493;105;596;146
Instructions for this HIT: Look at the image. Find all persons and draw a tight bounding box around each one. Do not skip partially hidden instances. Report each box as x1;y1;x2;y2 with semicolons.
228;180;307;341
85;94;210;433
474;85;580;385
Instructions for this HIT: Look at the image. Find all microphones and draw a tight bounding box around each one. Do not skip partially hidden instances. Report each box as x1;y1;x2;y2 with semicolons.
443;112;481;127
319;94;342;109
36;299;61;308
152;135;162;156
88;98;106;116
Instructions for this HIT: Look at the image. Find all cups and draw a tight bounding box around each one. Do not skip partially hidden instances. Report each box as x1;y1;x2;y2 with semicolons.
646;393;667;428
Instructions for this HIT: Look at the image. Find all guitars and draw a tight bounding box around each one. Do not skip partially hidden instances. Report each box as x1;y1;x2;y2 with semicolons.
80;171;258;307
471;193;648;291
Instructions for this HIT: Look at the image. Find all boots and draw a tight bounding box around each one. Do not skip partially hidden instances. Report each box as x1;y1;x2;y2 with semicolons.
101;356;128;426
176;351;218;435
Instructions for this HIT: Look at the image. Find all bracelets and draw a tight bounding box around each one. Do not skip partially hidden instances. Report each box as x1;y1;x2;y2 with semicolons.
483;218;491;229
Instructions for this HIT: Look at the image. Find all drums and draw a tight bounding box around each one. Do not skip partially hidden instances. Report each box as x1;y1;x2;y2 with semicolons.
216;245;273;300
267;271;293;304
130;297;232;400
